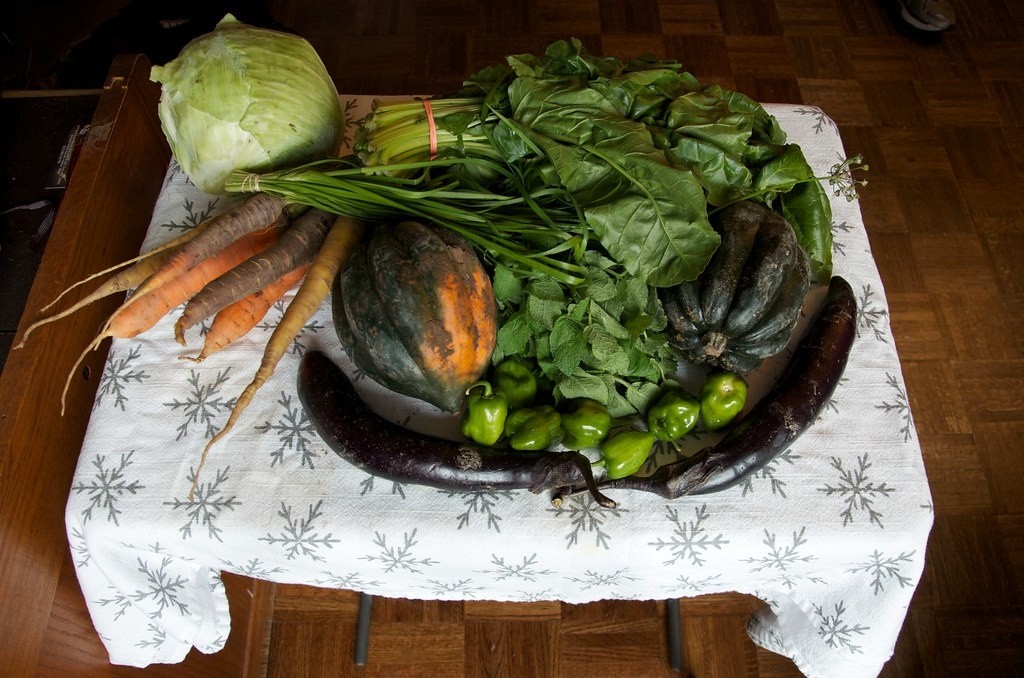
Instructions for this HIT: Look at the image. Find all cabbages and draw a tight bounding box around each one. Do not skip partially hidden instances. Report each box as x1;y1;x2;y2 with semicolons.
149;13;344;197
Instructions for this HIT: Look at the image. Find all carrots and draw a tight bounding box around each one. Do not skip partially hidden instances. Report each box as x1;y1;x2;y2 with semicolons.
13;191;355;498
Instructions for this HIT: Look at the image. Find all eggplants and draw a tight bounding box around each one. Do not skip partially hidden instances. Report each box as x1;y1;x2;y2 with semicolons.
297;350;617;508
551;274;857;509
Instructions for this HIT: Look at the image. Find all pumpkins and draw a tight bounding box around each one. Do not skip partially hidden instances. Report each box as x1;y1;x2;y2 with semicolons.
653;201;808;376
331;215;499;416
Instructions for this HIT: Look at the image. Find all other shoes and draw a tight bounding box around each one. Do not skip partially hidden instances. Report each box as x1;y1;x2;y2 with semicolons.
899;0;957;31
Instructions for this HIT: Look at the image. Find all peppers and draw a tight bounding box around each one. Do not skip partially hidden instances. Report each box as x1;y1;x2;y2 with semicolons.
458;359;747;480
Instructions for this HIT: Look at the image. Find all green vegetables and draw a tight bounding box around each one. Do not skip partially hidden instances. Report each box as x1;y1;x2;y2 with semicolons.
225;37;870;423
